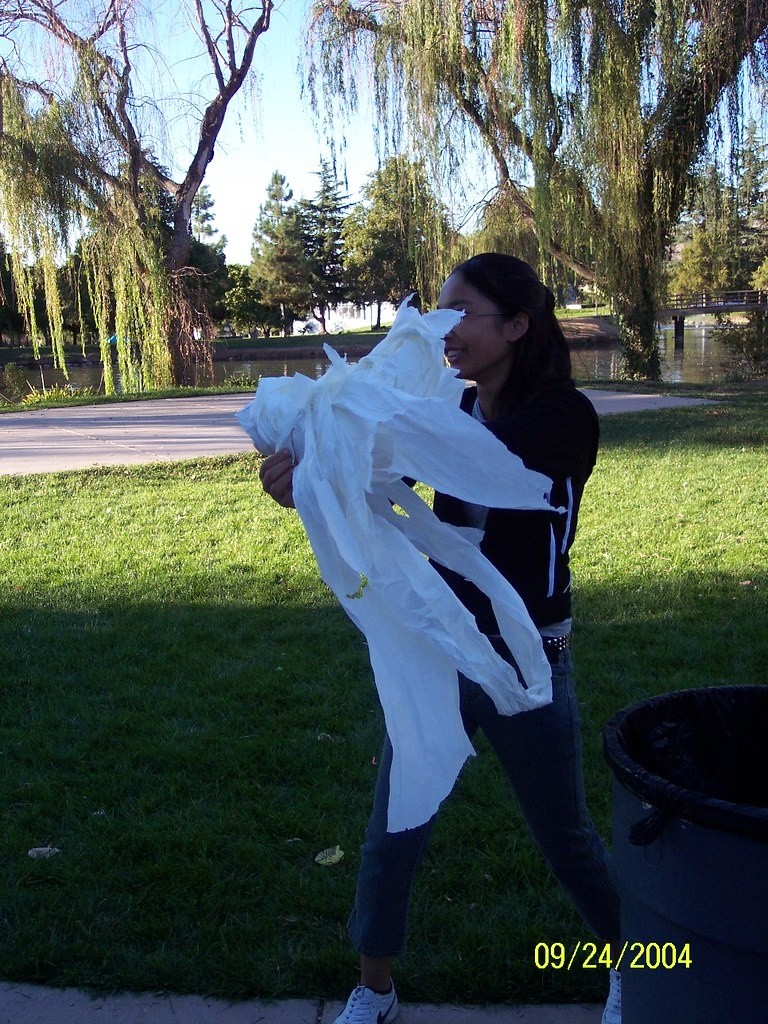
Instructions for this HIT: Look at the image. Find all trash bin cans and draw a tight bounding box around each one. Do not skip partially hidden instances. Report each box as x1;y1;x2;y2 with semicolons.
603;685;768;1024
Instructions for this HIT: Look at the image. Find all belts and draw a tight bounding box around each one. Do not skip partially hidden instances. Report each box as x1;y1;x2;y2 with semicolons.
547;634;570;651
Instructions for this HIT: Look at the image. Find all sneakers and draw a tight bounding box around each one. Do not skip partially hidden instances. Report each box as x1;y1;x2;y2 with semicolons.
601;968;624;1024
334;975;399;1024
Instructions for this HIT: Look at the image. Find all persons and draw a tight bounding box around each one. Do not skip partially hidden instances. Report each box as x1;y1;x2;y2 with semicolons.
259;253;621;1024
